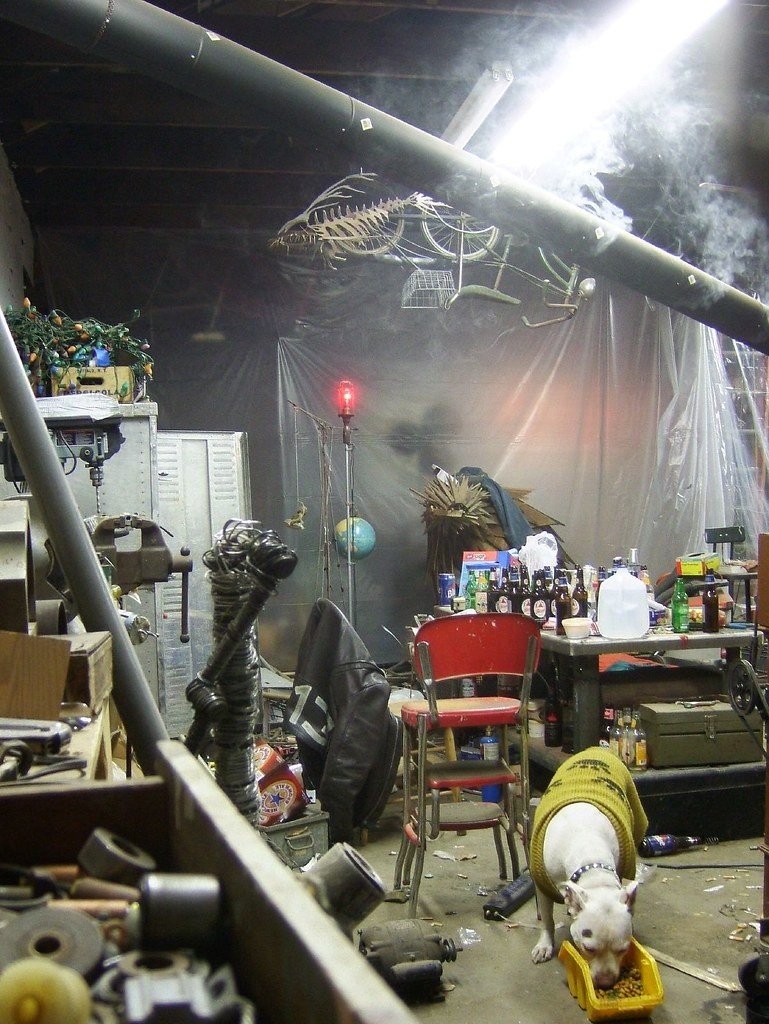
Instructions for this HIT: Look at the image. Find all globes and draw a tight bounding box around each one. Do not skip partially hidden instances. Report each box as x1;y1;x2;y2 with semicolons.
333;517;376;560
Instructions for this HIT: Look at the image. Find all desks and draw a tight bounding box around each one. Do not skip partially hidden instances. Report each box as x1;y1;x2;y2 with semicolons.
540;620;763;756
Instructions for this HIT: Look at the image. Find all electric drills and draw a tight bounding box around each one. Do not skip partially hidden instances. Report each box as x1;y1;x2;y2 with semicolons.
0;426;126;514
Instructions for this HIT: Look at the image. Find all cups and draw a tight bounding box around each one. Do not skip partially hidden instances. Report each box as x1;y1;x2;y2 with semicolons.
562;706;576;753
528;699;545;737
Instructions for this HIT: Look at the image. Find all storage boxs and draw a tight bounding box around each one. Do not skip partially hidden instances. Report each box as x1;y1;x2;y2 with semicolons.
49;365;135;404
257;807;329;867
41;631;115;714
638;700;762;769
458;550;519;602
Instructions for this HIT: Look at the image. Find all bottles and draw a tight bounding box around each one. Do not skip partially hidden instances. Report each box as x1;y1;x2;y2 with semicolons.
609;710;624;761
510;754;522;797
618;707;632;768
599;706;614;748
672;576;688;632
702;568;719;632
638;834;700;857
515;686;529;733
545;682;563;746
466;549;654;639
628;711;647;770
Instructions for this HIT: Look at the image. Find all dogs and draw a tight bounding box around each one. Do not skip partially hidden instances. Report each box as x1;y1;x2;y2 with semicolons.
529;746;660;992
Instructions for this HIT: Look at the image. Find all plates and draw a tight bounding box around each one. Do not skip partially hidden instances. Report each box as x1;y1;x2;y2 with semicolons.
669;607;725;627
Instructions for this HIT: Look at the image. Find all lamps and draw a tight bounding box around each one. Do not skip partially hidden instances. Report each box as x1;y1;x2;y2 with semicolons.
439;59;513;151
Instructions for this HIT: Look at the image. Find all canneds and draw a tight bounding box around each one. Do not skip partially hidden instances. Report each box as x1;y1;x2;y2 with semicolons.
438;572;456;606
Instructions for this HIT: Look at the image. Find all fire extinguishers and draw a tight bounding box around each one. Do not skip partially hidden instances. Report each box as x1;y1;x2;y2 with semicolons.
479;725;501;804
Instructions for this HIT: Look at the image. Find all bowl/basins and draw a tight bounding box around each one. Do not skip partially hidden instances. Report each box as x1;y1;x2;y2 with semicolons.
561;618;590;639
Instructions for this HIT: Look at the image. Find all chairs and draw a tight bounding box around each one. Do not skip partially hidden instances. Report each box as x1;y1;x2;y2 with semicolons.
705;527;746;560
393;612;545;922
